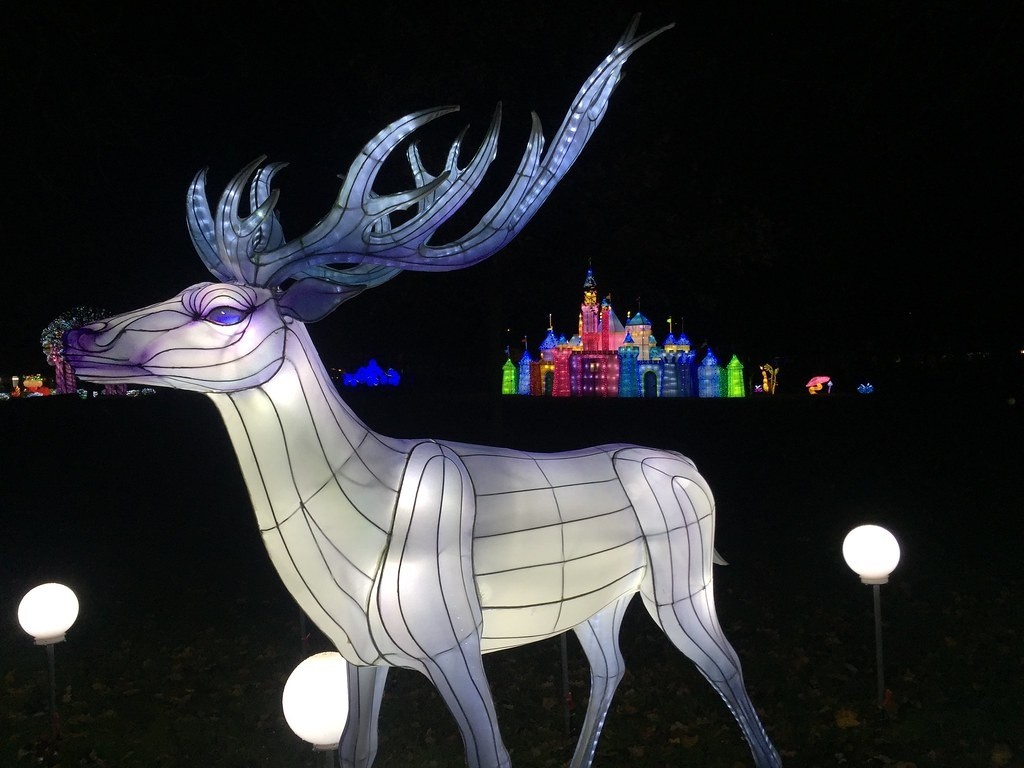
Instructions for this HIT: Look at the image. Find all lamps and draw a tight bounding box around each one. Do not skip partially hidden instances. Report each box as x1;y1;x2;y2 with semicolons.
842;523;903;586
282;652;353;752
17;583;80;647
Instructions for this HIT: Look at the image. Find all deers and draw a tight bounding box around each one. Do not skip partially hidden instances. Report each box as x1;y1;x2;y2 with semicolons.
58;11;786;768
